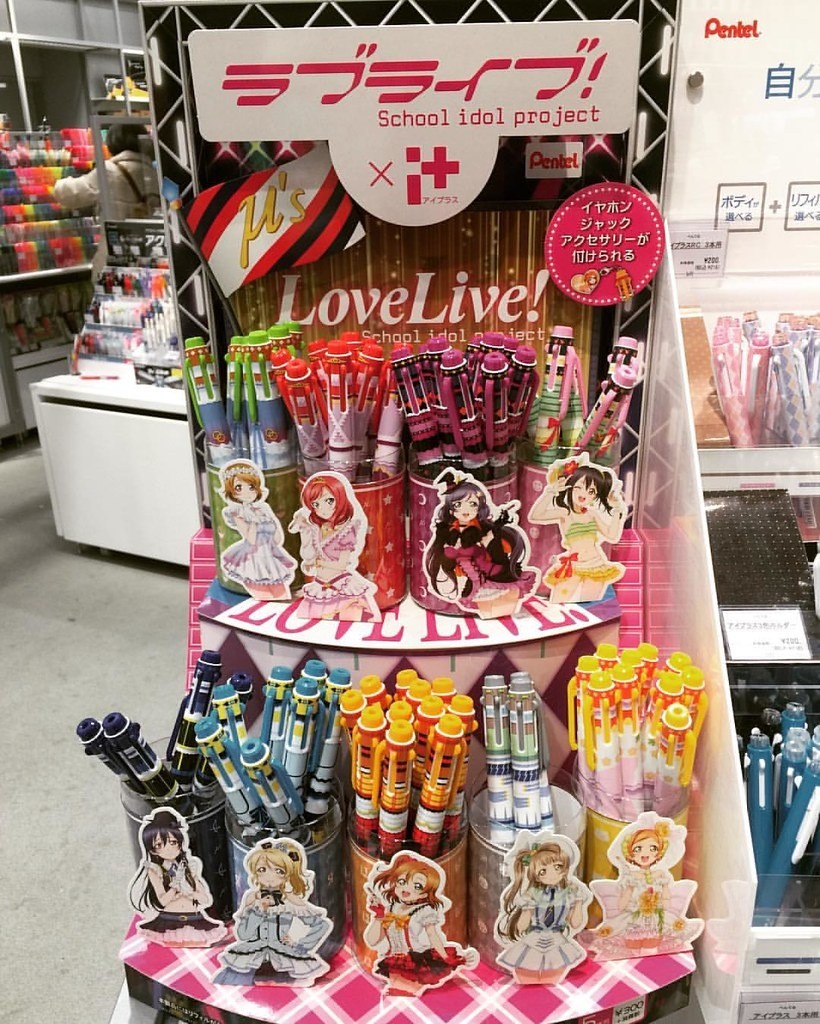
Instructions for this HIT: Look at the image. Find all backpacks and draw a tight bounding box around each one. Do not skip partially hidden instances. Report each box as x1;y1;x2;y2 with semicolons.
133;194;170;218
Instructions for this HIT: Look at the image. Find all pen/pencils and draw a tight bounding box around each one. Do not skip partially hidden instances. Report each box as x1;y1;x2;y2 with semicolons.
180;321;308;590
194;658;359;849
268;327;411;490
337;668;481;861
709;306;819;445
78;298;179;364
735;700;820;927
475;672;556;831
1;281;94;348
93;267;173;298
566;642;709;825
390;327;540;484
73;650;236;809
526;323;643;473
0;127;114;278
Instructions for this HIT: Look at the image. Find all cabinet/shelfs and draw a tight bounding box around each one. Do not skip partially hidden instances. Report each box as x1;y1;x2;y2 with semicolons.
638;215;819;1021
125;0;697;1024
0;129;111;441
29;114;200;567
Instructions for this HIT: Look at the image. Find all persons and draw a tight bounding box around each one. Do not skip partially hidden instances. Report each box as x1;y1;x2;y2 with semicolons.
53;124;161;288
